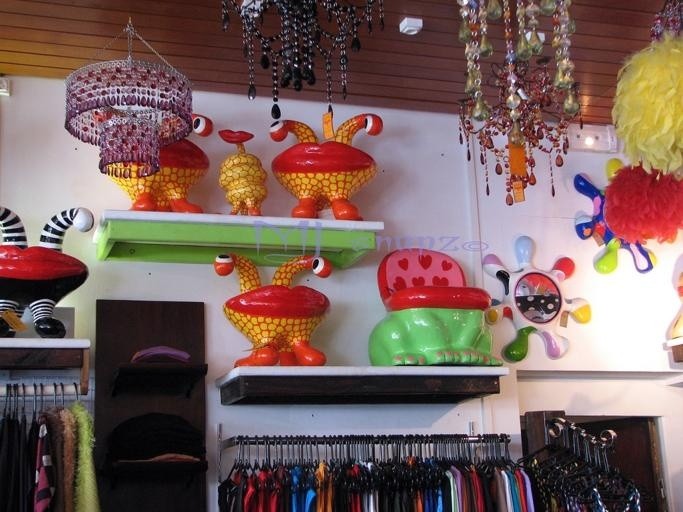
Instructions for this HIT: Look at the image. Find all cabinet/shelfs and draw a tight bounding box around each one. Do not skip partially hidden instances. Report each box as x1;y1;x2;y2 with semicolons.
94;208;511;407
94;298;209;512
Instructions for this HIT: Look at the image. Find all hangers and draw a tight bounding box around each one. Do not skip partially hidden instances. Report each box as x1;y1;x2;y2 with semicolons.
1;383;79;431
